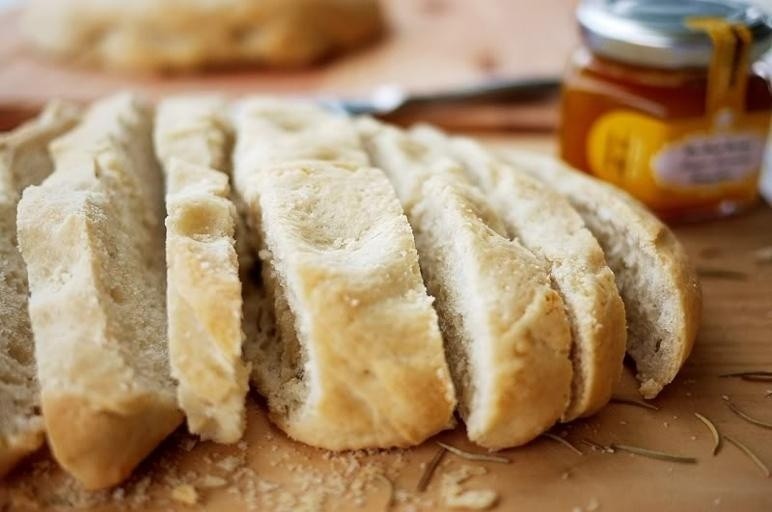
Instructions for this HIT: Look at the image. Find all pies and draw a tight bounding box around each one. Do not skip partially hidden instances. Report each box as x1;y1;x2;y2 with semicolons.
23;0;383;76
1;91;704;492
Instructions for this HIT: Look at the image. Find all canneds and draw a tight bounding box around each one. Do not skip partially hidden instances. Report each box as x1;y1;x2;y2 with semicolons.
556;0;772;228
